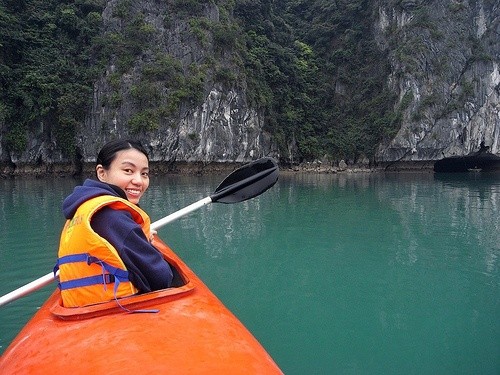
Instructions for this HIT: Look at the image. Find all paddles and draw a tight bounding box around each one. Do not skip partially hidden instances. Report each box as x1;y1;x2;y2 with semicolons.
0;156;280;304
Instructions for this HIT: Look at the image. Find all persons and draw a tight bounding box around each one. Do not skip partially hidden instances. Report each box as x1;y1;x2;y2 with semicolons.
55;138;174;308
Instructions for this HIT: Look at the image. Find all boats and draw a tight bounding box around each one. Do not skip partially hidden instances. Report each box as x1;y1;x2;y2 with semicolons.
1;229;287;374
